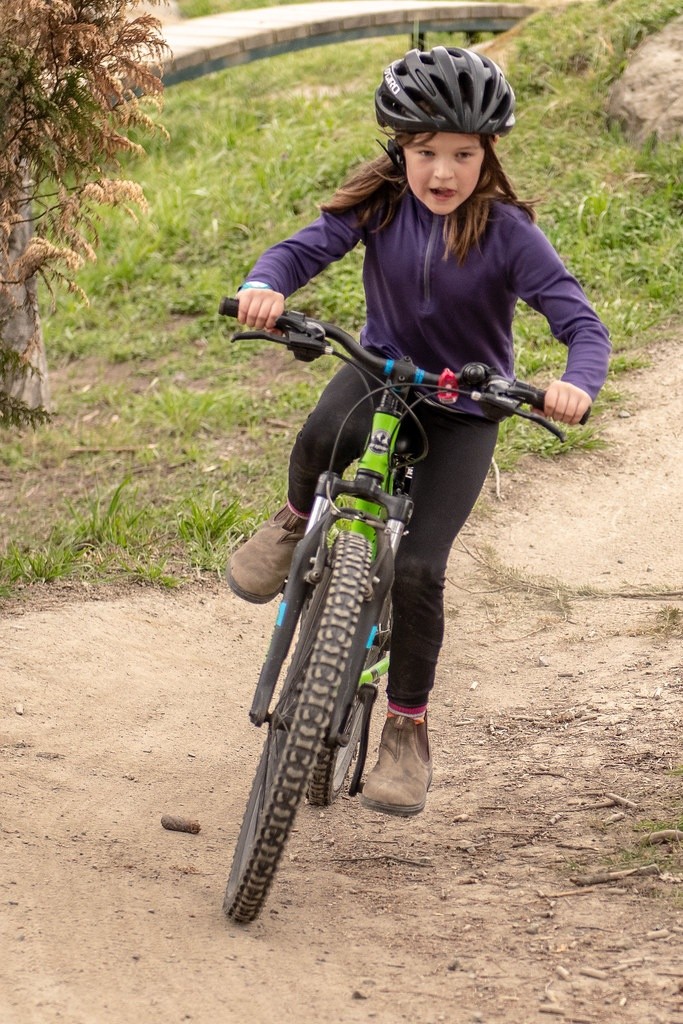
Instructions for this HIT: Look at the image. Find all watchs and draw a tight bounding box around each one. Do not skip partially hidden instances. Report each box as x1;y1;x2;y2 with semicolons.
242;282;270;288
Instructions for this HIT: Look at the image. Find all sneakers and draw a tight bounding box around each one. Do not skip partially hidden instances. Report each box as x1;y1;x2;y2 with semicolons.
361;710;431;815
225;500;308;604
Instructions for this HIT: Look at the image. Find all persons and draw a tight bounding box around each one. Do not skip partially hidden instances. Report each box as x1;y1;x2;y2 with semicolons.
228;45;612;815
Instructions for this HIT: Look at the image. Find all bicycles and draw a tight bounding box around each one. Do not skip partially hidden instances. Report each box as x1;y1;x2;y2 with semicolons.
220;293;594;927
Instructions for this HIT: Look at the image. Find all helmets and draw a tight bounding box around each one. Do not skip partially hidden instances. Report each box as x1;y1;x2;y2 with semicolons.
375;47;516;137
